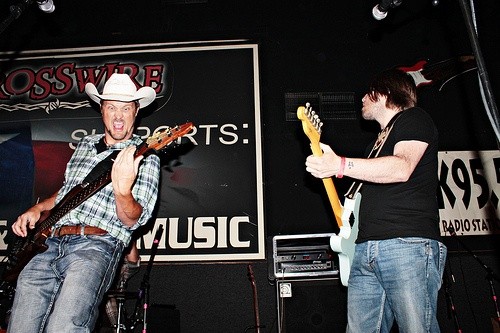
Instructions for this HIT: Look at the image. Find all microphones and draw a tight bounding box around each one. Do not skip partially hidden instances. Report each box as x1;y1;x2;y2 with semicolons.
372;0;402;20
36;0;55;13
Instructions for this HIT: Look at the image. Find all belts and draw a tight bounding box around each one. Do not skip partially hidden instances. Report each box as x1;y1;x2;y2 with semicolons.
51;227;106;236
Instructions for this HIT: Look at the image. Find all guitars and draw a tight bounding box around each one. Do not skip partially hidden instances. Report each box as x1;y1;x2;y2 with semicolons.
0;123;194;296
297;101;361;286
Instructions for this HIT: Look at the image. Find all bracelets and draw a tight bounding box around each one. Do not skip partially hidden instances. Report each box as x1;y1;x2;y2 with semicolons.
336;156;346;178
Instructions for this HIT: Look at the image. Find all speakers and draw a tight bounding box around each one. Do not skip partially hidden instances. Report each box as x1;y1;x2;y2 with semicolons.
277;279;348;333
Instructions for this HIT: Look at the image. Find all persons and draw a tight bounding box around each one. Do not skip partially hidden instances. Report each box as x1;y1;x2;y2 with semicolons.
6;74;160;333
305;67;447;333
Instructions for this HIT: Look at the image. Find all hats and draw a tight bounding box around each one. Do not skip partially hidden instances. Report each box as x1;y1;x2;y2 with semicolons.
85;73;156;108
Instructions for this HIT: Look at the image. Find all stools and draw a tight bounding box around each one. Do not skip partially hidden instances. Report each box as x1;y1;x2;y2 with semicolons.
105;291;138;333
